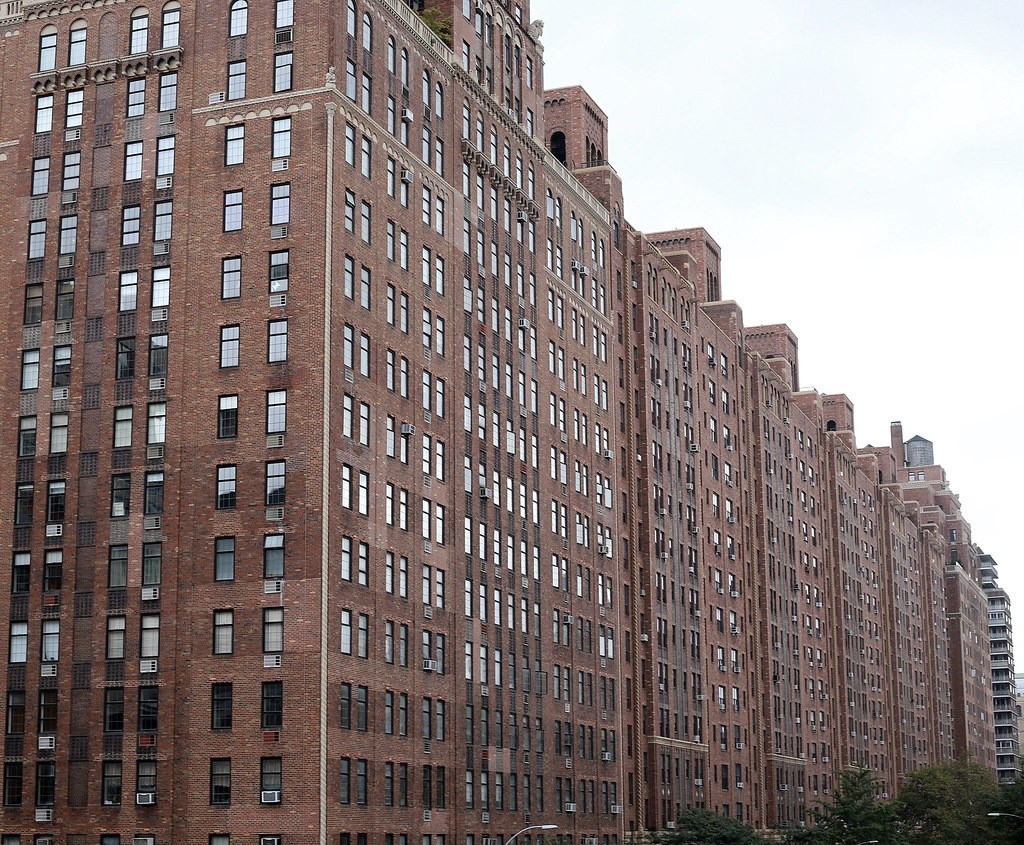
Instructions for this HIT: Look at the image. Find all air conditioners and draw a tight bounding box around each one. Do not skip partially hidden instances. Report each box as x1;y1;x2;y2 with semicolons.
137;793;155;804
402;108;416;122
570;261;589;276
36;839;53;845
423;660;438;671
563;615;573;623
598;280;953;830
585;838;599;845
518;319;530;328
517;211;527;222
262;791;281;804
261;837;281;845
132;837;154;845
480;488;492;498
400;424;417;436
565;803;577;811
482;838;497;845
403;170;413;183
